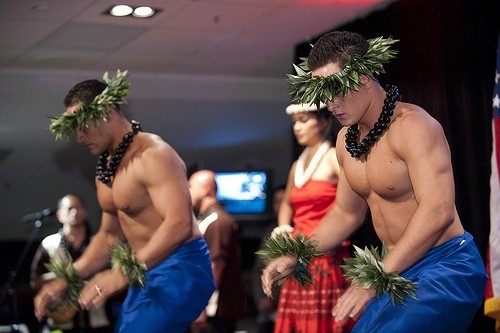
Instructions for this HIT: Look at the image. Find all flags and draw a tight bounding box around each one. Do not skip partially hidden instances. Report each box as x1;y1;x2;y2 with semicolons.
482;48;500;331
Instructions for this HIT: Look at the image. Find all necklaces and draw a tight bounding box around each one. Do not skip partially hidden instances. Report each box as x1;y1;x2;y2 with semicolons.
295;141;331;189
96;120;140;184
345;83;402;158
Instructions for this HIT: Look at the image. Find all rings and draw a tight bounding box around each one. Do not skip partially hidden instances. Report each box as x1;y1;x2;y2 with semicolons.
95;284;101;294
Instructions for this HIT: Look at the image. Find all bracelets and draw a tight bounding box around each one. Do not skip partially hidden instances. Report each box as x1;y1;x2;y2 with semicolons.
44;248;87;304
109;240;150;287
272;224;295;239
255;231;326;290
340;244;421;307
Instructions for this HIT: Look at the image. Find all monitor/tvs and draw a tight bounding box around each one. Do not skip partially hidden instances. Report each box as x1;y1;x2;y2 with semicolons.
212;167;273;221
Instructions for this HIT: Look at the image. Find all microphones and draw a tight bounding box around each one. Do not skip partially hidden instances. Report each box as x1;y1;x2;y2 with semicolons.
21;207;56;223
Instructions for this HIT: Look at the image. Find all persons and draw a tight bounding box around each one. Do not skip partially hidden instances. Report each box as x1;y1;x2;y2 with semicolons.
33;70;217;333
263;31;489;333
188;170;244;333
272;90;358;333
30;193;117;333
